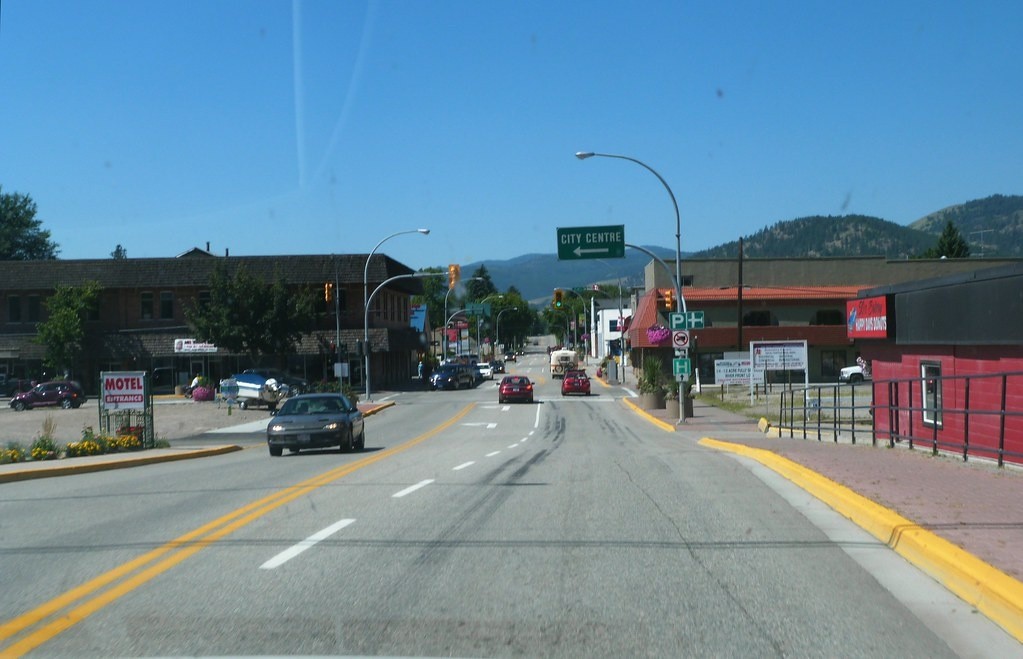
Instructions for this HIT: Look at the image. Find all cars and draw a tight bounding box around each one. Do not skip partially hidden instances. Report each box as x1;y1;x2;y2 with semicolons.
503;351;518;363
516;349;524;356
561;369;592;397
266;393;365;456
429;362;481;389
488;360;510;373
495;375;537;402
477;363;495;380
838;365;873;386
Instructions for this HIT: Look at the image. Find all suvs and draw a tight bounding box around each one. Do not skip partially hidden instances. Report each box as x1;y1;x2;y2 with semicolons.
245;369;310;393
8;380;87;409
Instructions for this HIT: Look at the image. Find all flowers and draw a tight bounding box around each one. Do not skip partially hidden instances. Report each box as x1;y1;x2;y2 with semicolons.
646;324;671;344
582;334;590;339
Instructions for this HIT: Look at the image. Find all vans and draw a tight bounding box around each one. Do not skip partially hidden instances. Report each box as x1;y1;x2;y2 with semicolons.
551;348;583;378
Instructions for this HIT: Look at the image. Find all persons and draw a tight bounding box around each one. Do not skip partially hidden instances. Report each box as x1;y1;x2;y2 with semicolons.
418;361;424;379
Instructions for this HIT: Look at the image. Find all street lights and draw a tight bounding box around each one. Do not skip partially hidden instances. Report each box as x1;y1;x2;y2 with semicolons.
577;150;707;434
360;228;431;403
556;289;590;367
475;295;504;364
496;307;518;353
443;276;484;366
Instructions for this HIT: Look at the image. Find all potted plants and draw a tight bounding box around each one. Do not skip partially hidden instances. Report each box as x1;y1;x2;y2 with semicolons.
636;355;696;418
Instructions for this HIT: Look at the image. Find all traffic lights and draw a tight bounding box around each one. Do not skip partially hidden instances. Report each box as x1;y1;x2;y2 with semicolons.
555;289;565;311
325;283;333;302
330;343;337;357
341;343;349;357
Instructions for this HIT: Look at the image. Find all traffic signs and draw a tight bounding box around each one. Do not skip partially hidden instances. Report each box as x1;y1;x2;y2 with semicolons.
557;225;628;261
